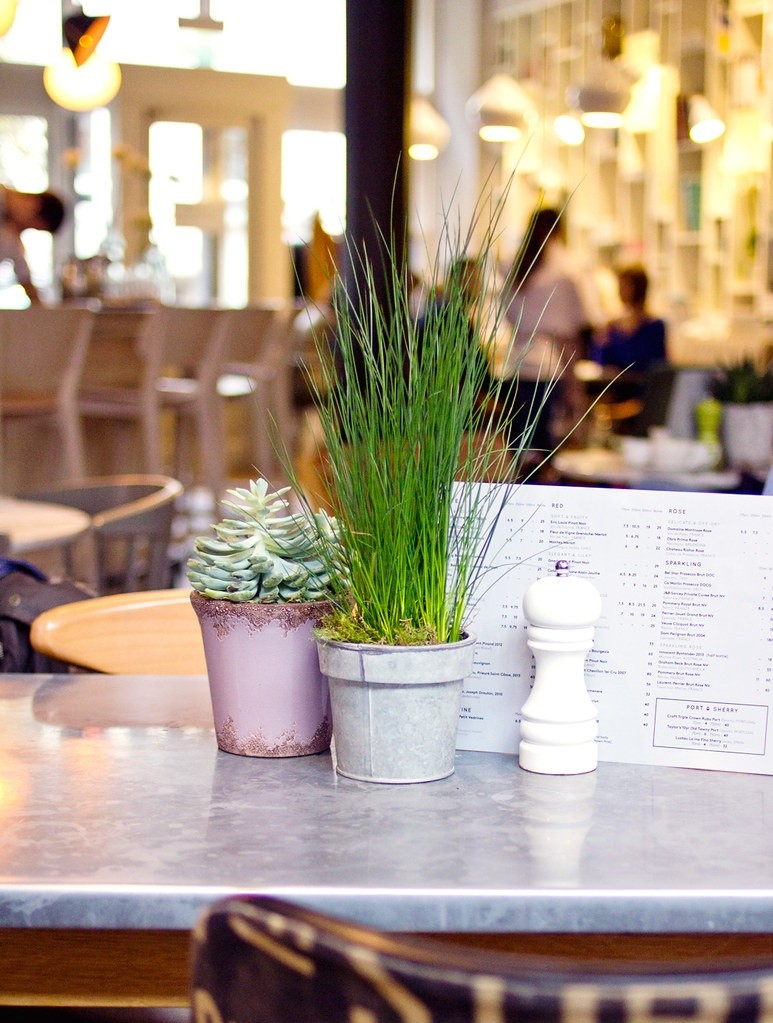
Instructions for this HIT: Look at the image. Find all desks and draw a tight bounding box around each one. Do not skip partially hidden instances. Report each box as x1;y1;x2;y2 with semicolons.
0;671;773;1023
551;447;739;491
0;494;90;554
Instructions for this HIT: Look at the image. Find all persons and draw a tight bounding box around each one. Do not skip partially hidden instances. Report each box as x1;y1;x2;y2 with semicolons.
502;206;601;466
419;258;492;390
0;186;65;308
591;268;665;365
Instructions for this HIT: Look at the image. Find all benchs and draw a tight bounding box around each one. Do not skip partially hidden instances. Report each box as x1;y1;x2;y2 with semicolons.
0;298;278;585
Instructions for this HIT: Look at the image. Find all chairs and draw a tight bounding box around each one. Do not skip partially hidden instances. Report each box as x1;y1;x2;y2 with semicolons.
16;474;184;596
188;895;773;1023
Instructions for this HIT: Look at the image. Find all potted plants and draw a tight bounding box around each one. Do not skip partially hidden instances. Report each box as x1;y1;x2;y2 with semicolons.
186;476;363;757
245;124;637;784
702;350;773;469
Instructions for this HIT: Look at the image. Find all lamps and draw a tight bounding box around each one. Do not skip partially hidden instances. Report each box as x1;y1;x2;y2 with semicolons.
579;57;632;128
409;94;450;160
62;0;111;68
686;94;725;144
465;73;537;144
